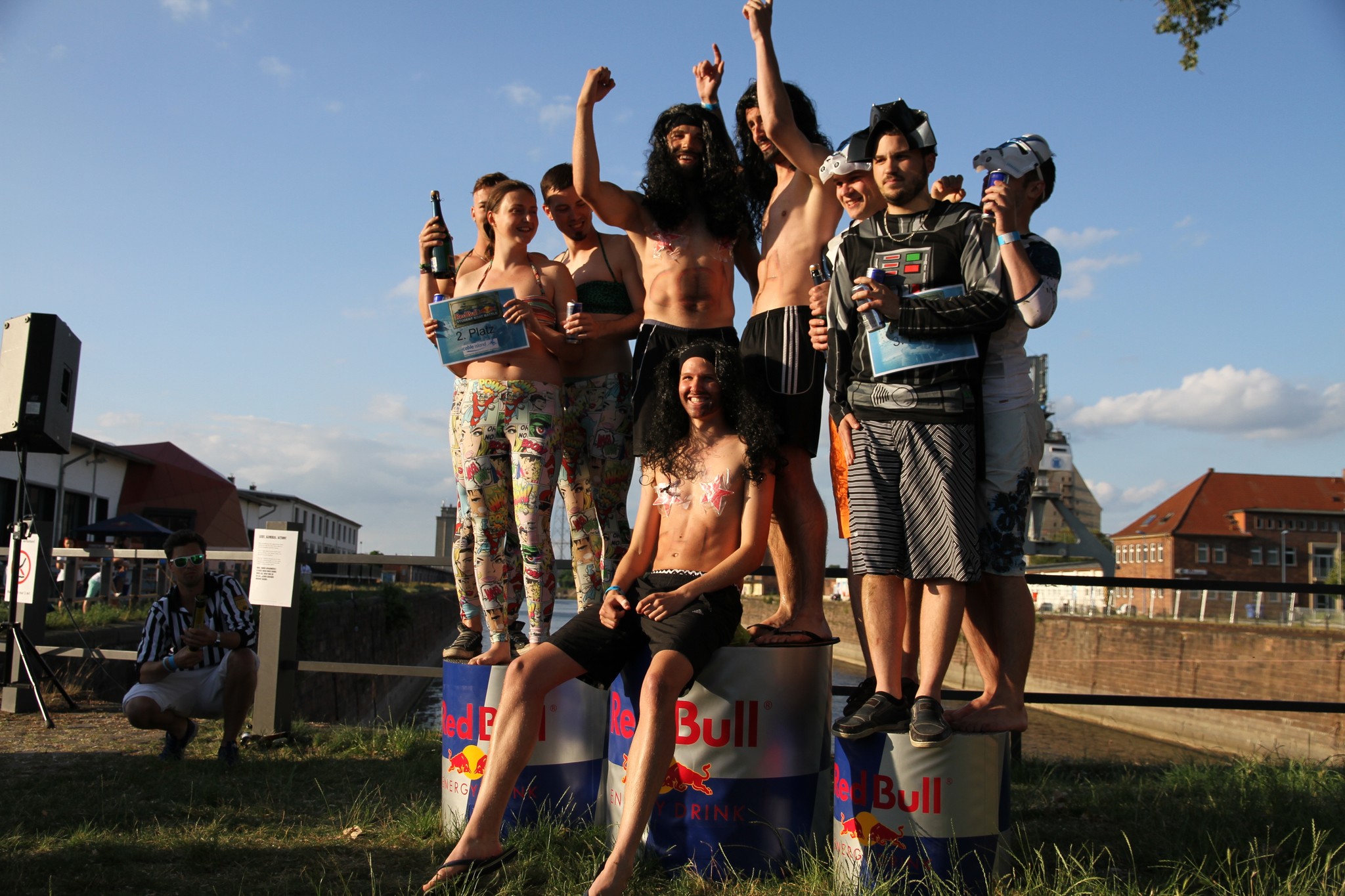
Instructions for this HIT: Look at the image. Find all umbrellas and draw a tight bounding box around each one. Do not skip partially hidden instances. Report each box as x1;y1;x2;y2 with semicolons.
73;511;175;536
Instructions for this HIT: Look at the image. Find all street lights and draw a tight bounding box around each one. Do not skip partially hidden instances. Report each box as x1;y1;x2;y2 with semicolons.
1281;530;1290;623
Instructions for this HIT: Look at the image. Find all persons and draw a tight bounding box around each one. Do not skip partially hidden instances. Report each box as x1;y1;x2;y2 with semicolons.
806;97;1061;749
690;0;844;648
120;529;260;763
57;534;134;616
419;162;645;666
420;339;778;896
300;557;312;578
572;64;760;473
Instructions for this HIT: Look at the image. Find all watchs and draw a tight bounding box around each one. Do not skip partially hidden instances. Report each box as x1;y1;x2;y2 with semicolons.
209;631;221;647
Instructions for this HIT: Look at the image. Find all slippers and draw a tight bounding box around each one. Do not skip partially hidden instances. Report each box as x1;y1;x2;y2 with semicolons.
743;620;775;643
756;628;841;647
583;859;622;896
423;847;518;896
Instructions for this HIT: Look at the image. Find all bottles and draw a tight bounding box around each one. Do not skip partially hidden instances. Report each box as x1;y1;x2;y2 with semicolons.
809;263;828;359
431;190;456;279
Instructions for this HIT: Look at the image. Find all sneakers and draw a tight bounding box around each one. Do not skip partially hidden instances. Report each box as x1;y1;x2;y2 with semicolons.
832;691;908;739
506;621;532;656
899;678;920;707
442;622;483;657
909;698;953;748
842;674;878;719
158;717;199;765
216;741;241;775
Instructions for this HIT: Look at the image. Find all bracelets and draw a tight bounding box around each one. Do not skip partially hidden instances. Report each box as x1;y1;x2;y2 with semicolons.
162;655;180;673
996;231;1021;246
433;344;438;350
420;262;433;274
603;585;626;602
700;99;720;112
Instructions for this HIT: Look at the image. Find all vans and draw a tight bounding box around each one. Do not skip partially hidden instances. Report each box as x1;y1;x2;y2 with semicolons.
1120;604;1136;616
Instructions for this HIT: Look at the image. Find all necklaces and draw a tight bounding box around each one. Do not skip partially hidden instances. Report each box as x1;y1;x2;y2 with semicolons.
473;248;490;263
883;198;936;242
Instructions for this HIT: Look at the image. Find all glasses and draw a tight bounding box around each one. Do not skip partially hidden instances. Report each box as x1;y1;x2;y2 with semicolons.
171;554;206;568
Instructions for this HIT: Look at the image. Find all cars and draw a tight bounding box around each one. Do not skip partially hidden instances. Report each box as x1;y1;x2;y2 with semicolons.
1040;603;1053;611
1087;605;1098;613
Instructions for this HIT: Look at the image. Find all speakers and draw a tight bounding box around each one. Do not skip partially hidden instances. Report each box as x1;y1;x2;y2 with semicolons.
0;312;82;454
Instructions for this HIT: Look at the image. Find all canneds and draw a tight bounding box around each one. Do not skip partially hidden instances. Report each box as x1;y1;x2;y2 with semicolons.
981;169;1009;224
566;299;582;343
866;267;886;285
433;293;450;302
851;283;886;333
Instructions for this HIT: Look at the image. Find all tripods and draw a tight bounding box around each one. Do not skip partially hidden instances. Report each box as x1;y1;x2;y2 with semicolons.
0;447;80;729
